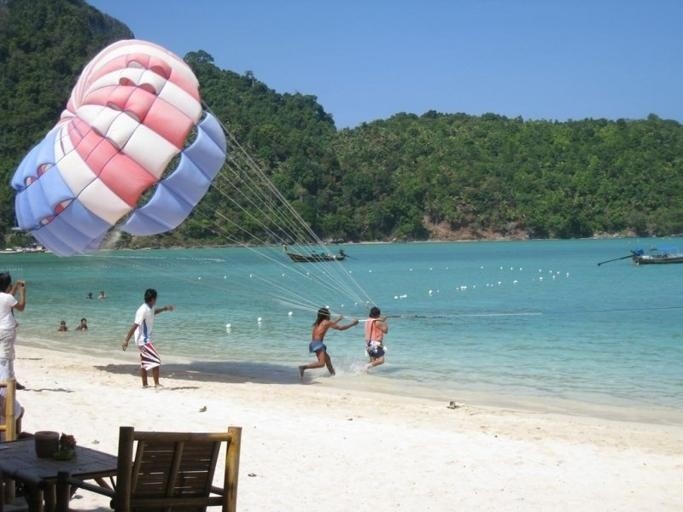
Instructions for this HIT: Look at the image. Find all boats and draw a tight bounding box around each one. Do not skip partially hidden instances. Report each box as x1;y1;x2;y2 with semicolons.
633;249;683;265
287;250;347;263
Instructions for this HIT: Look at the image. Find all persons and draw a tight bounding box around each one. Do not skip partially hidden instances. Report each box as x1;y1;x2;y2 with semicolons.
299;307;360;379
0;272;28;391
85;291;94;300
0;386;24;444
74;317;90;332
120;287;176;387
56;320;68;333
363;306;390;373
97;290;107;300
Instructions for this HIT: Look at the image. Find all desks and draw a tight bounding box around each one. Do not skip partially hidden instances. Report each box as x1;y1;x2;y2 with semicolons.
0;435;116;512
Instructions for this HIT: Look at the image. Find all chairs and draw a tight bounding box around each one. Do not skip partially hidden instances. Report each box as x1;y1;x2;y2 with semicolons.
111;427;242;512
